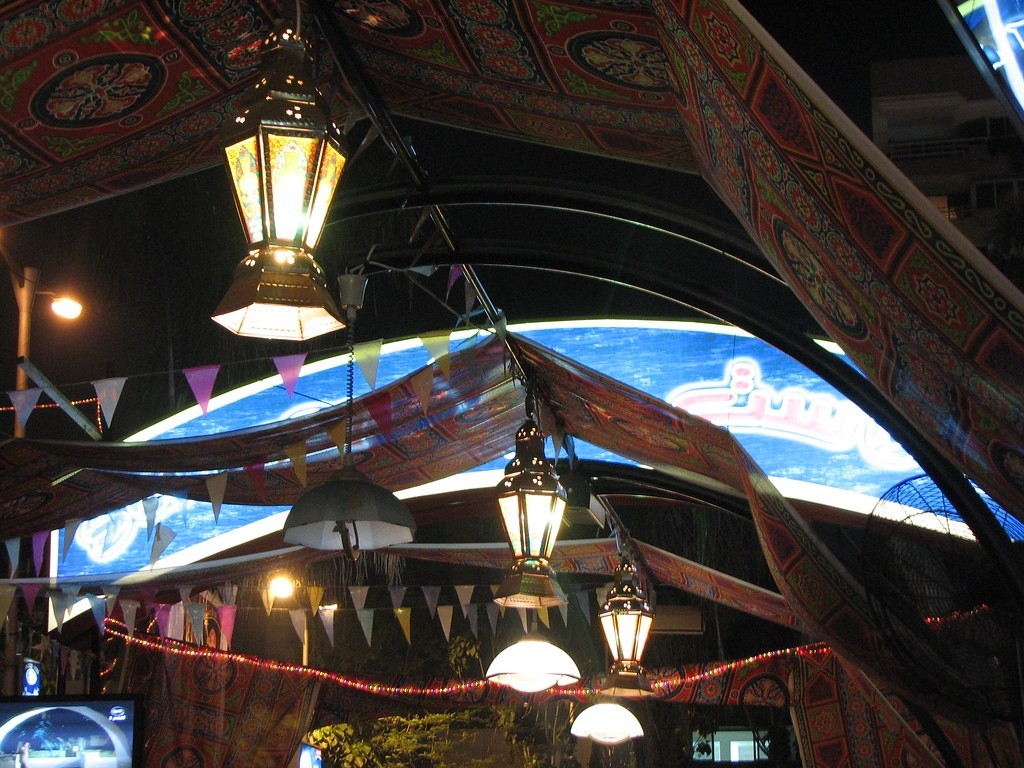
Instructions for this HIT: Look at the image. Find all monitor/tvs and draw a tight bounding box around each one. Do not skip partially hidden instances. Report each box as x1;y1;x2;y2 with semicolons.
21;655;43;697
297;741;323;768
0;692;144;768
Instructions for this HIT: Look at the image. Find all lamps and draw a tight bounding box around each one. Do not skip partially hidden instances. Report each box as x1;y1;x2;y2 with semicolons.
569;687;644;744
492;390;570;610
484;609;583;693
211;1;352;343
596;542;655;680
282;273;418;563
